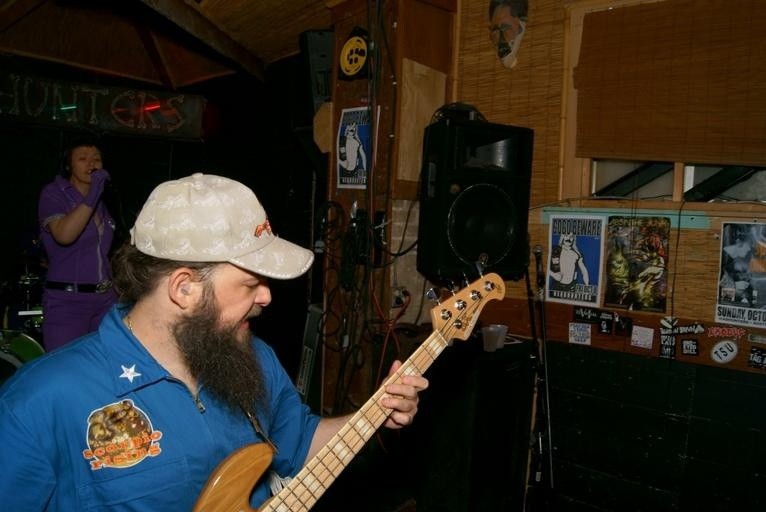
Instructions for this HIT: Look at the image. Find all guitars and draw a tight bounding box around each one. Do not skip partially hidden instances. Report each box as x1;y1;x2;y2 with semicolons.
192;262;506;512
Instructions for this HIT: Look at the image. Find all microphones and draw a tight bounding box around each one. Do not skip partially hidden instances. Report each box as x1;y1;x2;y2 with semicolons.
91;167;115;189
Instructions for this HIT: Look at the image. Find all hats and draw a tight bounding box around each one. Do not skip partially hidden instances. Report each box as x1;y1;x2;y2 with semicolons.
129;172;316;282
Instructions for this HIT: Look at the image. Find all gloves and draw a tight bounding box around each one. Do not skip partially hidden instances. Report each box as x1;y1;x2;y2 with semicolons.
80;170;112;209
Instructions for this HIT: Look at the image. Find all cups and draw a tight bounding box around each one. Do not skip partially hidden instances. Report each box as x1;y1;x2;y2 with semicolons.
481;324;508;351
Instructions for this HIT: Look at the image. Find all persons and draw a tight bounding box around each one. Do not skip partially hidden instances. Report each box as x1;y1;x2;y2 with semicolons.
0;167;433;511
35;133;120;352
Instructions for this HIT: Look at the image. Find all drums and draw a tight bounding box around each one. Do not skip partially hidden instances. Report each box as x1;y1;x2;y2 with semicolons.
0;329;46;383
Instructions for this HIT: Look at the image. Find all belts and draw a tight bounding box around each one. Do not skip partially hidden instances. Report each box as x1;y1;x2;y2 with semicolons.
43;278;116;295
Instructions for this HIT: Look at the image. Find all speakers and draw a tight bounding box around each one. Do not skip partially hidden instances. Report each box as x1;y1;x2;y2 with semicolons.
417;117;535;286
